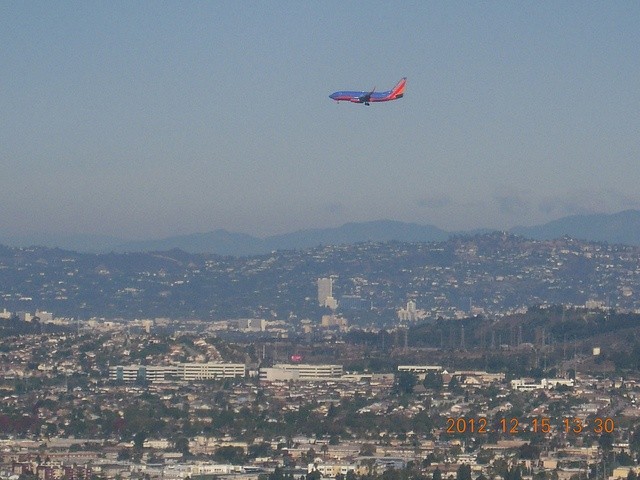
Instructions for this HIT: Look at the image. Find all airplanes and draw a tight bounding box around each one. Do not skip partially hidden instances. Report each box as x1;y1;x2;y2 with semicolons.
329;77;408;106
